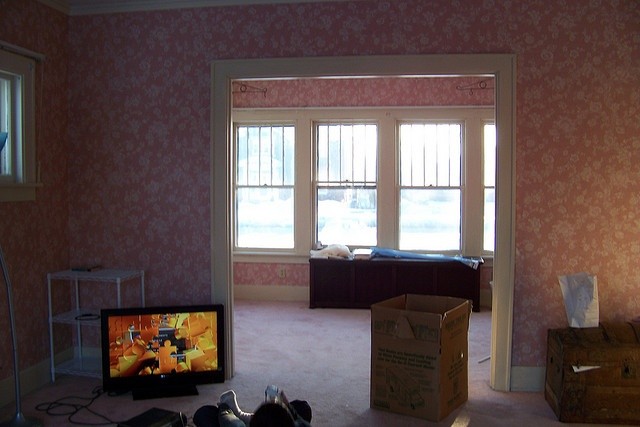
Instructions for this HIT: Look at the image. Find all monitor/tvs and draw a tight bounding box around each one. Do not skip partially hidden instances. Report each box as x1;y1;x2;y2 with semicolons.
101;304;225;400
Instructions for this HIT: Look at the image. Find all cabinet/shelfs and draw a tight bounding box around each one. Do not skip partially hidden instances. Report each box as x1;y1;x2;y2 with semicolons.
46;267;147;383
308;254;483;312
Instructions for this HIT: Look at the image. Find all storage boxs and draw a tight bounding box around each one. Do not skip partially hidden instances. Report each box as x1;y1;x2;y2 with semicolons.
544;321;640;425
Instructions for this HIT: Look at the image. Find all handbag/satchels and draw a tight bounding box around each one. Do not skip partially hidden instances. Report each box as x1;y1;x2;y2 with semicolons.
558;272;599;328
